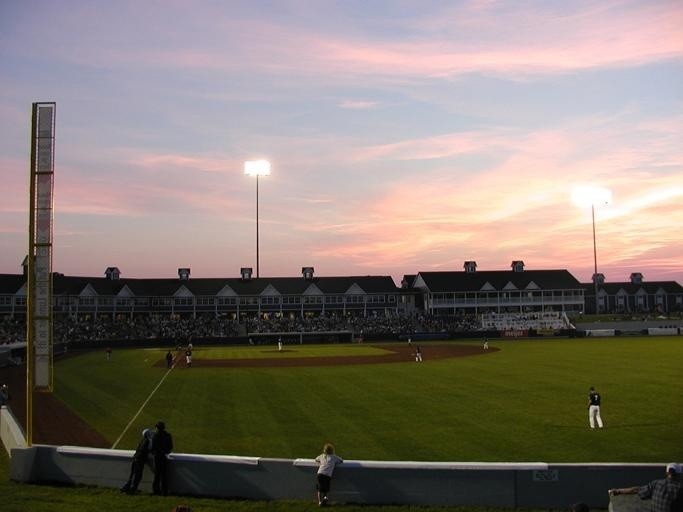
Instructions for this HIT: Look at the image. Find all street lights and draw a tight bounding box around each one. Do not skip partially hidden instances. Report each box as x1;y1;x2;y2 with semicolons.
242;157;270;278
570;182;614;313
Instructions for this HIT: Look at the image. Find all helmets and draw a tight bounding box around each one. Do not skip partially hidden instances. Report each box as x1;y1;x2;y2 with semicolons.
143;428;150;437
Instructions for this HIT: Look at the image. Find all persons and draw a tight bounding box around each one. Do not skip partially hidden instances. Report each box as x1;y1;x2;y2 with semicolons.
607;463;682;512
149;422;173;496
0;384;9;409
570;502;591;512
120;429;153;495
585;387;603;429
315;444;342;507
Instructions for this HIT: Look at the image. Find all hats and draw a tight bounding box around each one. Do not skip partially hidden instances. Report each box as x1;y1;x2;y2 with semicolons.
667;464;680;473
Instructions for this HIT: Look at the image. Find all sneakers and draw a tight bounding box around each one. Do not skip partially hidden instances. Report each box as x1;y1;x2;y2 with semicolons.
318;496;329;506
